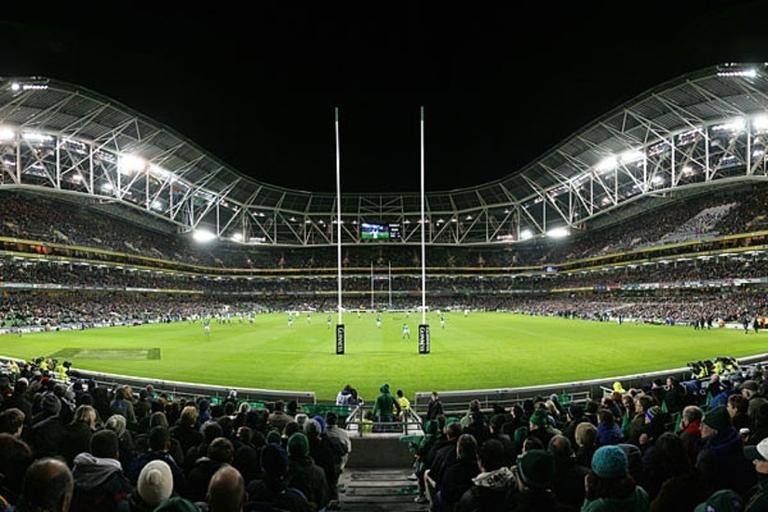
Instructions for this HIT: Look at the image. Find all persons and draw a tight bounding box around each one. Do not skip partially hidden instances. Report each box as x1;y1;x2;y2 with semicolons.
411;357;768;512
0;191;768;337
1;381;410;511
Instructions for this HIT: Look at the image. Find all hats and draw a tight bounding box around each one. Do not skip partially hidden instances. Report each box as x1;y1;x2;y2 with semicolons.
518;449;553;488
530;408;547;425
295;412;338;434
381;384;389;393
645;405;660;421
137;460;173;504
703;407;731;431
105;414;126;438
591;444;642;478
744;437;768;460
